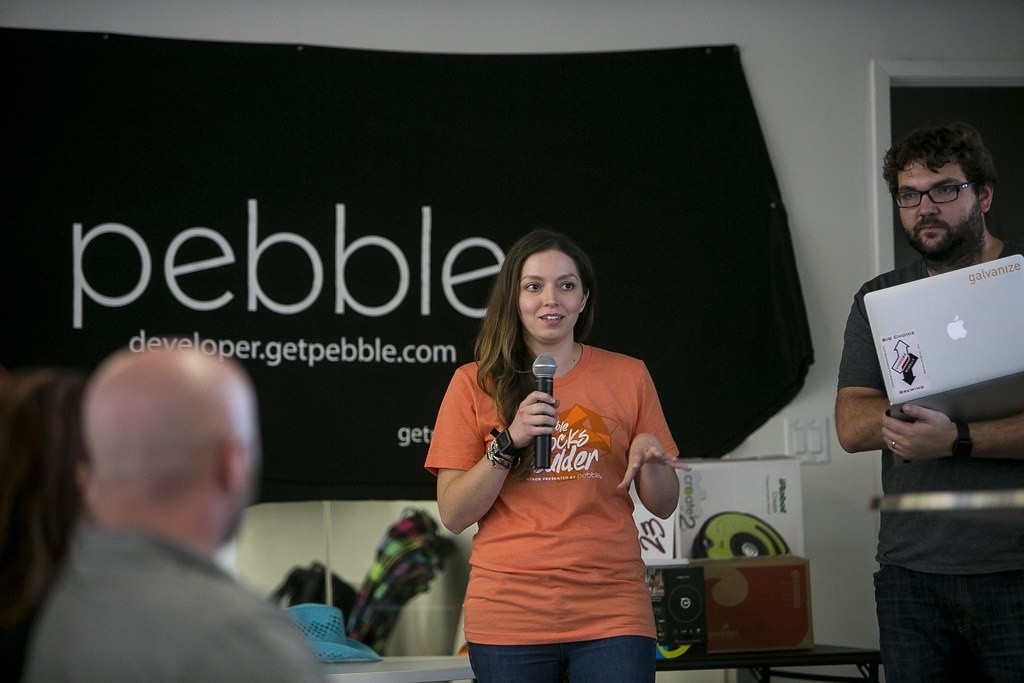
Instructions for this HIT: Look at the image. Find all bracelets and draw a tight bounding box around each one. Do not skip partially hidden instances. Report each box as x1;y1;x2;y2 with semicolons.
486;440;512;469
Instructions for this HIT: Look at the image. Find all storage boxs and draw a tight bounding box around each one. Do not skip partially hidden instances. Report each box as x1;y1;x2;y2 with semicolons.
645;563;707;658
626;455;808;561
690;555;815;653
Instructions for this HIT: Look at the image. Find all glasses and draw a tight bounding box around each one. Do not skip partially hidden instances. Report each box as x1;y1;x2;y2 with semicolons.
892;182;977;208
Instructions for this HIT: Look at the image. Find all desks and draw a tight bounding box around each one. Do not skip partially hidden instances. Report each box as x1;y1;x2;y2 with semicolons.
655;645;882;683
324;654;473;682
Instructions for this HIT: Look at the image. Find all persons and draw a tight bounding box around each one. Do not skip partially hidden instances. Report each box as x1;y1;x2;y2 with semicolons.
1;372;95;682
424;234;691;683
834;122;1024;682
25;343;322;683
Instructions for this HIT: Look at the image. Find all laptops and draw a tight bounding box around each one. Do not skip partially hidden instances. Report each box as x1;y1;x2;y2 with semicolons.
864;252;1024;467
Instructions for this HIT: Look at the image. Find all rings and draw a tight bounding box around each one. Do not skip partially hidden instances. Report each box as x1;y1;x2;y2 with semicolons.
892;442;895;448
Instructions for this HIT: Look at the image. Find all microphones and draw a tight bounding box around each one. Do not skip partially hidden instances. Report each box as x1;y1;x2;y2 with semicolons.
533;354;558;470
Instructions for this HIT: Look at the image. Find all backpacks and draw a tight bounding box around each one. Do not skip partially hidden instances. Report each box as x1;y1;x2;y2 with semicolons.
270;563;355;619
343;506;457;649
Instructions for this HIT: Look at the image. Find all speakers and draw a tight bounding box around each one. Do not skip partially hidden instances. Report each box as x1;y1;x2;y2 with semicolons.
646;566;706;660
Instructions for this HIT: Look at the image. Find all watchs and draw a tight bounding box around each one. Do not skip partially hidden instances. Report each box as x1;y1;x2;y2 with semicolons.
952;422;972;463
496;426;526;457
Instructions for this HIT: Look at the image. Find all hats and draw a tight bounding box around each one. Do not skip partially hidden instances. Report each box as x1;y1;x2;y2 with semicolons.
281;604;383;662
